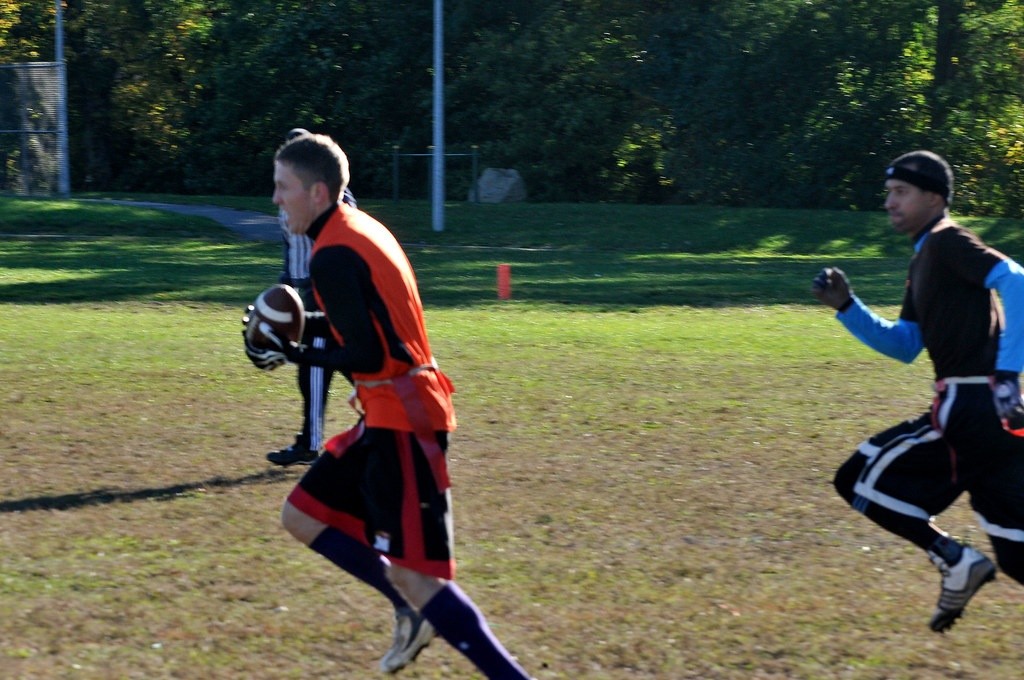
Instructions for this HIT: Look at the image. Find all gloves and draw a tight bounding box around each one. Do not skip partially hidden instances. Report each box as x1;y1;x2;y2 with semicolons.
811;267;854;312
241;308;253;334
996;373;1024;440
244;322;303;371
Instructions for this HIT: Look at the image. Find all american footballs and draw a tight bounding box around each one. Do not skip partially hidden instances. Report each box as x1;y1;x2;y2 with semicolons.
244;283;306;372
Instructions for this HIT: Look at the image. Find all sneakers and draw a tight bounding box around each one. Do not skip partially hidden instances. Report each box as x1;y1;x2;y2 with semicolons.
267;443;318;466
379;608;437;673
928;543;996;633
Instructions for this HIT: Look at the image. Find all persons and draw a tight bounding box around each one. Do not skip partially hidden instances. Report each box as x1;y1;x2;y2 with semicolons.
240;127;543;680
813;149;1024;633
